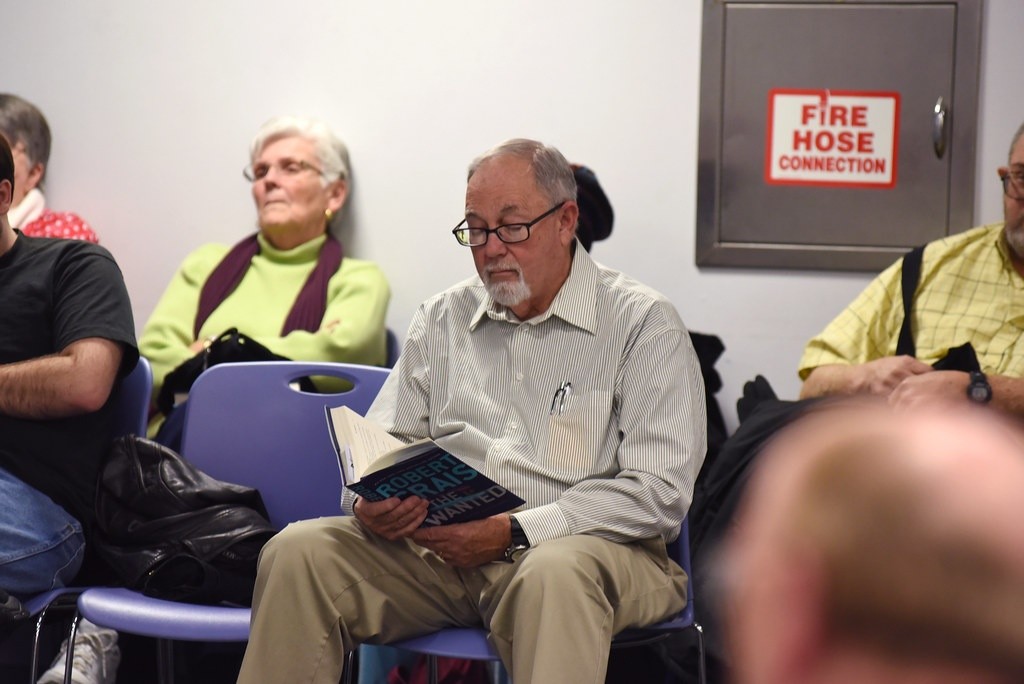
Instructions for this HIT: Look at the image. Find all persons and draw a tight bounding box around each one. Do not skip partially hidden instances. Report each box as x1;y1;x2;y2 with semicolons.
797;121;1024;406
234;136;711;684
0;136;143;599
37;117;393;683
0;93;102;244
680;396;1024;684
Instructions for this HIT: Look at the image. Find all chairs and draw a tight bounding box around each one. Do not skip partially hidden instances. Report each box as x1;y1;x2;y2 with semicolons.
64;361;392;684
0;354;153;684
400;514;704;684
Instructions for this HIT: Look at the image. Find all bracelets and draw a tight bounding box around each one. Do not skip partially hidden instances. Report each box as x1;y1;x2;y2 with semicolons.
204;336;215;349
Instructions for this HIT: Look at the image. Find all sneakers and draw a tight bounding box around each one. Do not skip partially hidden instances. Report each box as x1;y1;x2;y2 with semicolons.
35;617;120;684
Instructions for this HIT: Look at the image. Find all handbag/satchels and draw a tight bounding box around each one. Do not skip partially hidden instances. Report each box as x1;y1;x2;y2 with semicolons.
84;433;279;607
161;329;314;414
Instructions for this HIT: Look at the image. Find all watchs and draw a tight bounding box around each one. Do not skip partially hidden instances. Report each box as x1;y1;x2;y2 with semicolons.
967;369;993;406
503;515;529;563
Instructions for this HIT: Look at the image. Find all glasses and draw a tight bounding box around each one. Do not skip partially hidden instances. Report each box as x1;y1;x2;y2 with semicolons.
244;159;328;181
452;203;564;247
1001;171;1024;200
